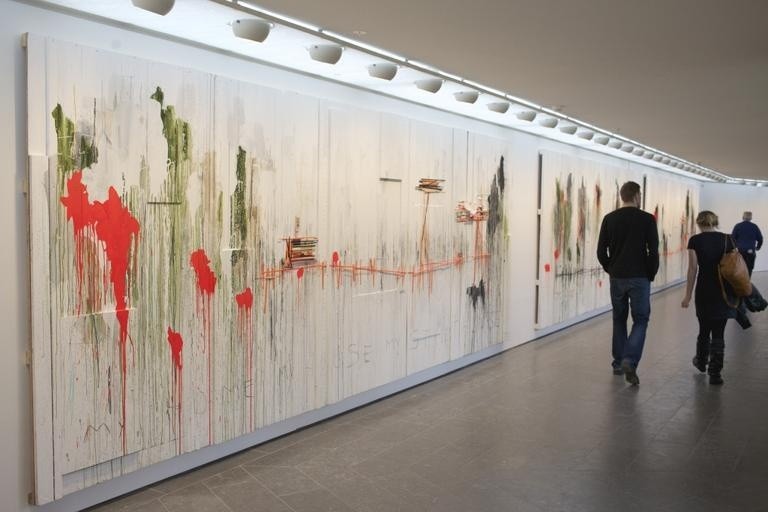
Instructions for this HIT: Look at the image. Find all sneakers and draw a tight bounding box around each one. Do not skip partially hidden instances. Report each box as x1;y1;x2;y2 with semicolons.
693;355;723;385
612;359;640;384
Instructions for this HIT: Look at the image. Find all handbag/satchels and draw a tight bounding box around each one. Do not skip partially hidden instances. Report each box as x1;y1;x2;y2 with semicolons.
719;231;754;298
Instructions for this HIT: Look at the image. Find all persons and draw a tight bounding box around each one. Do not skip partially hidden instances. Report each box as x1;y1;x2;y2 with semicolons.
730;211;764;280
679;209;739;387
596;181;660;385
